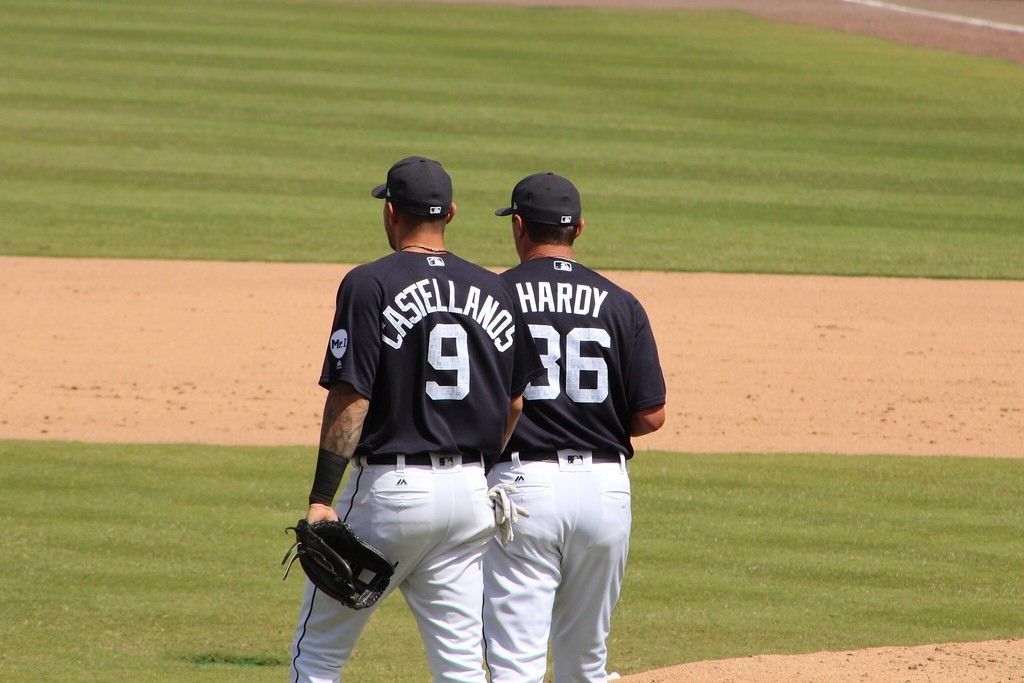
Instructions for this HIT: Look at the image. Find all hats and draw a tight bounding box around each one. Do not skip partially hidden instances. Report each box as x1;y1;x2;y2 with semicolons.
495;171;580;226
371;155;452;217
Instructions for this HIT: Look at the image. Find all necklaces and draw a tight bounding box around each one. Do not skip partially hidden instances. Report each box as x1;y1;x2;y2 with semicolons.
399;245;450;253
528;253;577;263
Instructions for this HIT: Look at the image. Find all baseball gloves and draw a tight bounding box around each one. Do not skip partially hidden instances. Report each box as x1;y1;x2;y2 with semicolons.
294;517;395;611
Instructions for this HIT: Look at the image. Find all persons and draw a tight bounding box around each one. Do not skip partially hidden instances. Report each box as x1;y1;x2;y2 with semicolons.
291;157;545;683
483;171;667;683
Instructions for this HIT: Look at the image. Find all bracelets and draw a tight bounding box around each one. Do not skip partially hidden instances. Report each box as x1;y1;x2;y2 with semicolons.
309;448;350;506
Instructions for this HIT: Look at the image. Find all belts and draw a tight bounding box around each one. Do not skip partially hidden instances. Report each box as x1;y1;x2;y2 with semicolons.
365;450;481;466
494;451;621;463
